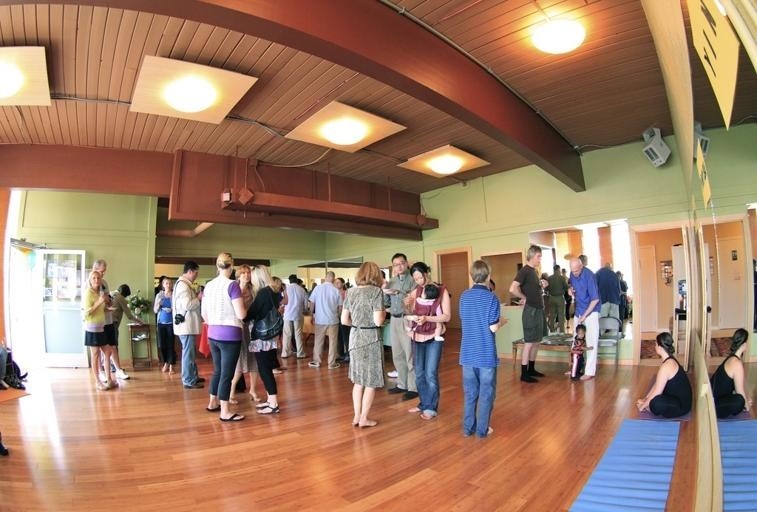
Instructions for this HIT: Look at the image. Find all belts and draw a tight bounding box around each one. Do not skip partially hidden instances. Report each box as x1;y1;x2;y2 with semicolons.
350;325;380;329
391;313;407;318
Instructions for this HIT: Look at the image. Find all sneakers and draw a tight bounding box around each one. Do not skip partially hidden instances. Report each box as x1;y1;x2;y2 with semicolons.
387;371;399;378
95;364;130;391
328;363;341;369
309;361;320;368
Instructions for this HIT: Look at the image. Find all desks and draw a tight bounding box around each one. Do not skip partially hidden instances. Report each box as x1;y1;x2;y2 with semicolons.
512;338;572;373
129;325;151;368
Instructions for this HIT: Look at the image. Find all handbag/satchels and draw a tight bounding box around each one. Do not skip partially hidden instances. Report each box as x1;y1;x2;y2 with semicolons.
255;307;284;341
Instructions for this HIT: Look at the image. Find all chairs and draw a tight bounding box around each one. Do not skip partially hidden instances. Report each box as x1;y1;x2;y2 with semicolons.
598;316;625;367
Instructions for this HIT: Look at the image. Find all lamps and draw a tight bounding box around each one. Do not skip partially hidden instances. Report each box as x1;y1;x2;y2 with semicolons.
532;18;587;55
0;45;52;107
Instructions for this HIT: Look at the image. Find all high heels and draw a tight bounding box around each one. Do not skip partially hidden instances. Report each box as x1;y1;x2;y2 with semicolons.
249;392;262;403
229;397;239;406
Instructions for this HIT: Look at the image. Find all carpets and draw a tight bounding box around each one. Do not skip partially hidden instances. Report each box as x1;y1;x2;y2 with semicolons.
0;387;31;403
718;419;757;512
628;374;692;420
708;373;752;421
712;337;733;358
568;419;681;512
640;340;662;359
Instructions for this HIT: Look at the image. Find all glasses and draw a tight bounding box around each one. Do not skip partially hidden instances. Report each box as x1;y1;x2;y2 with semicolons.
393;262;407;268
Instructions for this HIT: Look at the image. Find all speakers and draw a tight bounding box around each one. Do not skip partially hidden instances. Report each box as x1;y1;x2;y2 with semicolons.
642;134;672;168
693;130;711;159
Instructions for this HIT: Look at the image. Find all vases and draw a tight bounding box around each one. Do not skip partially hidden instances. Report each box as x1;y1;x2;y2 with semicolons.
136;315;143;322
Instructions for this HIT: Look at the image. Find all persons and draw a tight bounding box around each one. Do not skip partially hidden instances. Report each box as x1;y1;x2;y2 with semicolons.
84;259;132;383
457;260;509;440
540;254;631;337
709;327;752;420
81;271;117;392
405;283;445;342
508;245;548;382
341;262;387;428
565;256;601;379
381;253;418;399
274;269;350;369
201;251;283;422
0;432;10;456
153;260;205;389
569;324;594;380
489;277;497;292
401;260;453;421
100;283;144;372
633;330;691;418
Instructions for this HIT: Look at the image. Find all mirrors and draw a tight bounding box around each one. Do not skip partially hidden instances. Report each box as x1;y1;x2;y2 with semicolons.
296;256;364;308
679;0;757;512
529;220;632;340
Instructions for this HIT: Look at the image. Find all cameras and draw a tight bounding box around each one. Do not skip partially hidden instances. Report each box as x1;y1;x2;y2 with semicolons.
174;314;184;325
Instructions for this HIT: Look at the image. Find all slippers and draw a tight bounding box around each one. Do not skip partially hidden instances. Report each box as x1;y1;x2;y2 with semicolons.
219;414;245;422
206;405;222;412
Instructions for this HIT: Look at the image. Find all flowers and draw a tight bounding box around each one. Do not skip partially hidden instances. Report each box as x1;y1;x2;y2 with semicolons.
127;290;152;315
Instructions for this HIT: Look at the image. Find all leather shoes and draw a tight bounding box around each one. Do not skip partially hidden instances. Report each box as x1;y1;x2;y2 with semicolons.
196;378;205;382
184;383;205;389
388;388;418;400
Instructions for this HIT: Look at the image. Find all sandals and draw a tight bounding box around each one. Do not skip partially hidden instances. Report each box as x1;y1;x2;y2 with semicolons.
256;405;280;415
255;401;271;409
352;417;377;427
